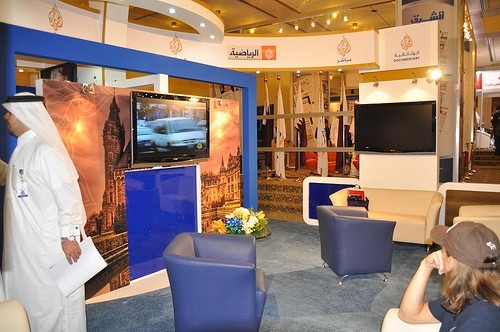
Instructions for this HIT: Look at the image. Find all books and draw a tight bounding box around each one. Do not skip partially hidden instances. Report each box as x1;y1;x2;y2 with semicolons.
47;236;109;298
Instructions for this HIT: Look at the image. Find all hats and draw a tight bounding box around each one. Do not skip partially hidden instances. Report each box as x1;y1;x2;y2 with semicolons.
430;221;500;269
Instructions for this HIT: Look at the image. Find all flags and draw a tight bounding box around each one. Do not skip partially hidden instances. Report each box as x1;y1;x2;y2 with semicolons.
261;70;352;179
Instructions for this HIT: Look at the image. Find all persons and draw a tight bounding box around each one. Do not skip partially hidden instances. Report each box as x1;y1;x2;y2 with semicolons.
0;91;87;332
397;220;500;332
478;123;495;148
491;106;500;151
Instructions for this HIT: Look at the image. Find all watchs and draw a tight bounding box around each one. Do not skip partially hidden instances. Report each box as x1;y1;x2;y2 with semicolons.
61;236;77;243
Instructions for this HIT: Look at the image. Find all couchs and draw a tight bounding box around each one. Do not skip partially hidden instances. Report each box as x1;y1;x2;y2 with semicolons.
163;232;265;332
316;206;396;284
329;187;443;253
453;204;500;241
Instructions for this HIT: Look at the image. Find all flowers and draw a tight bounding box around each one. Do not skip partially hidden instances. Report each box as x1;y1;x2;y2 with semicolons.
206;207;272;239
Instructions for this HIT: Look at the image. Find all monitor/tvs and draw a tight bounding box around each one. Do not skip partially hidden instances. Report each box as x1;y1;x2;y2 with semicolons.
129;90;210;168
354;100;437;155
